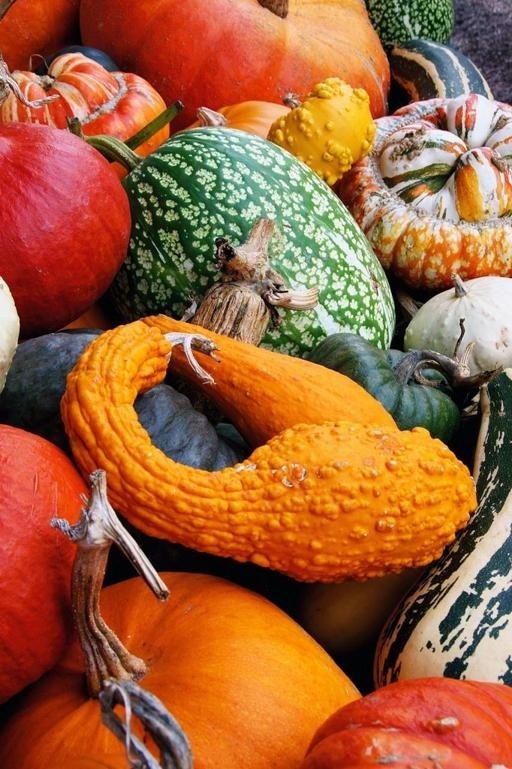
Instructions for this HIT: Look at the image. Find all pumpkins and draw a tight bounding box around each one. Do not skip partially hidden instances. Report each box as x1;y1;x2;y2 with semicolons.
1;1;512;769
